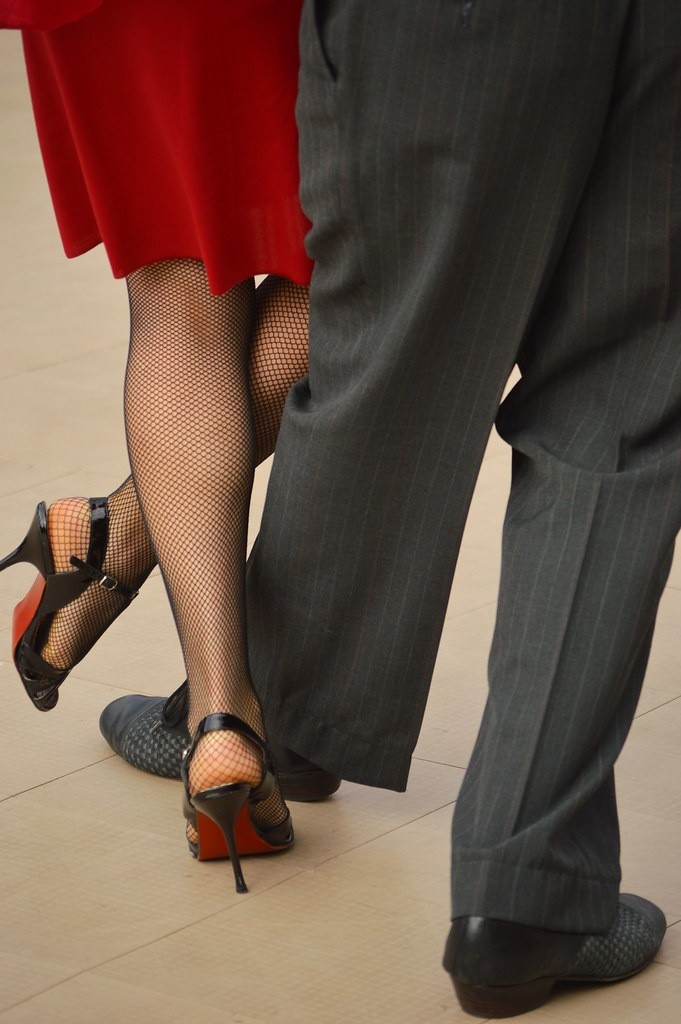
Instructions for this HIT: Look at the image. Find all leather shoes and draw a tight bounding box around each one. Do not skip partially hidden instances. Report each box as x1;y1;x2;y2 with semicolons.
441;892;667;1017
100;692;342;799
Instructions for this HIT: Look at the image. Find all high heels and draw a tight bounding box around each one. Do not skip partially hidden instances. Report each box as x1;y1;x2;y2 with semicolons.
0;496;113;713
180;713;296;891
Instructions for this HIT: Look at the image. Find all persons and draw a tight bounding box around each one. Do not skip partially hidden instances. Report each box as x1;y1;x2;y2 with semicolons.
0;0;310;894
100;0;681;1019
